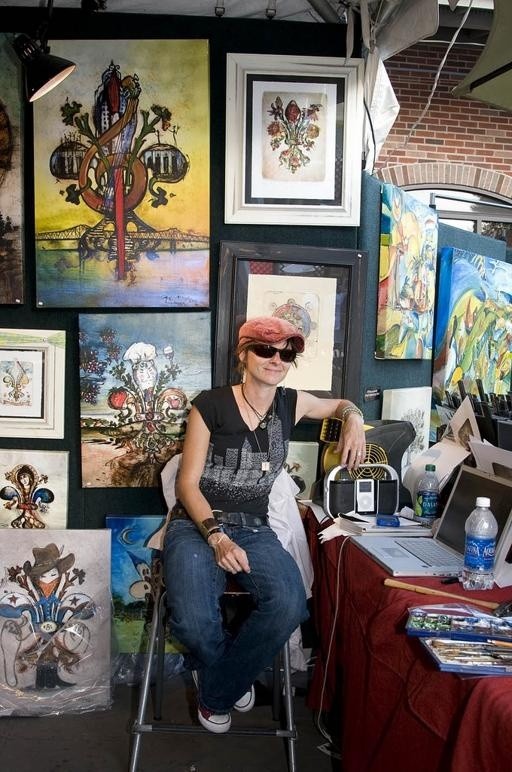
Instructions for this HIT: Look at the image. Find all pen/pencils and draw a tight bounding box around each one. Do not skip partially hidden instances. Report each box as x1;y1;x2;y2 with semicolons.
487;639;511;648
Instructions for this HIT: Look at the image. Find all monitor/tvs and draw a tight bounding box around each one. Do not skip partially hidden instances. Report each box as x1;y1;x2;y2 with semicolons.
350;464;512;577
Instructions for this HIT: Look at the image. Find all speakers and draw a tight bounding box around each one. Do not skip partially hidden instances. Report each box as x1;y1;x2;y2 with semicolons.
375;480;399;516
329;480;354;518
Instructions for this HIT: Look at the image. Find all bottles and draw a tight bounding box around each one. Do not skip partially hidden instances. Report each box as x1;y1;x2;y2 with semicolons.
464;497;500;595
414;464;439;525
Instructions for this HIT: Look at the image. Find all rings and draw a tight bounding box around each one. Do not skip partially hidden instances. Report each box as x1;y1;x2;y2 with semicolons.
355;449;361;457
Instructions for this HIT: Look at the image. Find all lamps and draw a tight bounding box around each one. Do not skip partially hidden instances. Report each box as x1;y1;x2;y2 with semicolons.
4;0;80;108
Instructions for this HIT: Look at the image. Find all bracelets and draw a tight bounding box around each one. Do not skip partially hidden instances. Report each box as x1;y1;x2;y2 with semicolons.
341;404;364;422
200;517;223;539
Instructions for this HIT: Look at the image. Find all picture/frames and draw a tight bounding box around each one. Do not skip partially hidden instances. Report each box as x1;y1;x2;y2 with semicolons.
205;231;370;444
216;46;365;232
0;324;70;443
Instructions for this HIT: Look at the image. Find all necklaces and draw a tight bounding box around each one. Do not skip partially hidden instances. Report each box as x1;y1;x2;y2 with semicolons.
240;382;277;474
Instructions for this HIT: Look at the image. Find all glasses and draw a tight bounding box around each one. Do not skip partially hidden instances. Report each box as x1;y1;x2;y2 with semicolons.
246;343;297;363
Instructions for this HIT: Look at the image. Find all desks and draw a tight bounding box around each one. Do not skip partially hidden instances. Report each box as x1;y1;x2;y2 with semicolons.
295;492;510;770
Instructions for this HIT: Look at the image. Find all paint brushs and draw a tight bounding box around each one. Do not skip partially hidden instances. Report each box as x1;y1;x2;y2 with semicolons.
431;639;511;663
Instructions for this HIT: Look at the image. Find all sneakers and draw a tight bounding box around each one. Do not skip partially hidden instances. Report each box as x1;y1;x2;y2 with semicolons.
232;684;256;714
191;668;232;734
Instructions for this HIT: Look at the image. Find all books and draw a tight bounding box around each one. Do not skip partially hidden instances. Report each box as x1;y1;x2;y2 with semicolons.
334;509;436;538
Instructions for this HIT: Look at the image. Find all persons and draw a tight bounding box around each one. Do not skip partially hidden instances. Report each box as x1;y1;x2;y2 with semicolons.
147;312;368;736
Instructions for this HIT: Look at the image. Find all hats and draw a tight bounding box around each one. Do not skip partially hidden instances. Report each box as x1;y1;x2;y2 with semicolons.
237;315;305;355
22;543;76;579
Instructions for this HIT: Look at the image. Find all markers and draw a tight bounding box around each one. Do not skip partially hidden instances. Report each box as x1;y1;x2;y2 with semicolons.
440;576;464;586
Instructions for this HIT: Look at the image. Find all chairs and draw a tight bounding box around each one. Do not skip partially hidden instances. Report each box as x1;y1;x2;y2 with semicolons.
116;448;310;770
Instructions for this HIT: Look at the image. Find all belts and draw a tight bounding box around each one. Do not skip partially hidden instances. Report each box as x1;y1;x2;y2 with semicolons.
168;508;268;528
